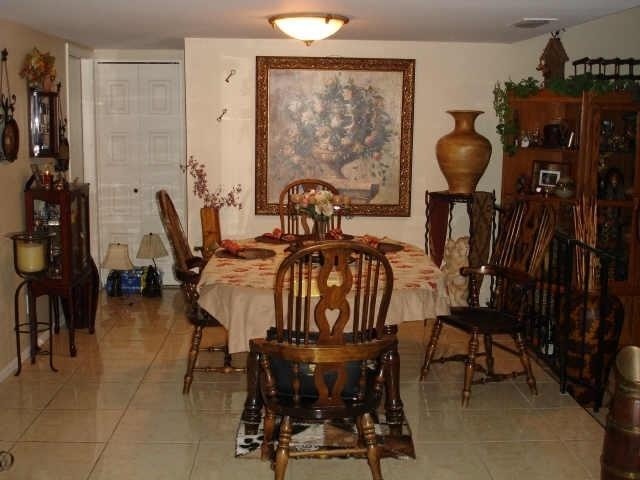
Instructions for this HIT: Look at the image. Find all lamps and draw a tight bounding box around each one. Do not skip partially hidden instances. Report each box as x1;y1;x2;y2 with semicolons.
268;12;349;47
136;233;169;297
99;243;133;297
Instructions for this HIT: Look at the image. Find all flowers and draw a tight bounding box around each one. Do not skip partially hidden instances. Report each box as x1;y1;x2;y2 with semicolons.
19;47;56;81
179;156;242;209
291;189;339;220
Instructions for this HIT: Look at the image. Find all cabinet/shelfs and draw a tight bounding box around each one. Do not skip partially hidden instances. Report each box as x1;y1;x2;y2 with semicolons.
10;232;59;376
500;88;640;394
25;183;99;357
423;190;495;326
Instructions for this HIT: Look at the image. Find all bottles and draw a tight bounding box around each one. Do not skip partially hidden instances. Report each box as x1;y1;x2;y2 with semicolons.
514;129;530;147
107;264;156;297
43;165;52;190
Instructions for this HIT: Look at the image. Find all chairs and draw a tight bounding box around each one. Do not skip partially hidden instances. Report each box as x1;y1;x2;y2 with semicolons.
156;190;247;394
250;241;397;480
419;196;556;409
279;179;340;236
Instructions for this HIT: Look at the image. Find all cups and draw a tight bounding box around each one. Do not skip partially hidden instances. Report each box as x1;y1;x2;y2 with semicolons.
528;128;540;146
565;127;576;148
543;123;560;147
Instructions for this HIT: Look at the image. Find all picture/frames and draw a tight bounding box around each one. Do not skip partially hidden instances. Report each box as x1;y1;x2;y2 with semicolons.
255;56;415;217
28;83;61;157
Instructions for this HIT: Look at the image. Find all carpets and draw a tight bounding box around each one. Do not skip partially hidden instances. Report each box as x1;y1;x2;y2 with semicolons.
234;388;416;464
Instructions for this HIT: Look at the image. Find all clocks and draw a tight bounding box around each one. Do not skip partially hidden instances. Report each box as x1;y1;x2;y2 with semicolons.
0;48;19;162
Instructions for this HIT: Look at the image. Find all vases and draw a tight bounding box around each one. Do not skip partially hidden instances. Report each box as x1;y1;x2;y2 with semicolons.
200;208;221;259
314;221;326;242
435;111;492;196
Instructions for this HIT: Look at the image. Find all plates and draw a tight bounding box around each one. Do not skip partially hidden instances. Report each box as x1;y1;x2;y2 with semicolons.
376;243;404;253
293;251;355;265
255;233;303;245
309;232;353;241
215;247;275;259
